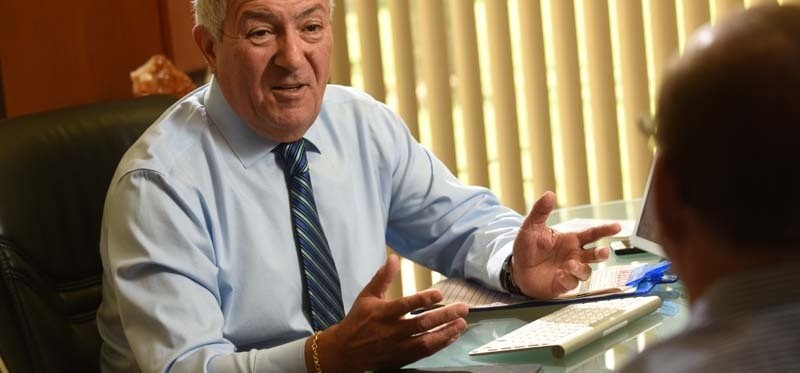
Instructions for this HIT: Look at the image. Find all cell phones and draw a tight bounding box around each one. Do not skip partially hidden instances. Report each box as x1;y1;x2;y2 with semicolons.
611;239;648;255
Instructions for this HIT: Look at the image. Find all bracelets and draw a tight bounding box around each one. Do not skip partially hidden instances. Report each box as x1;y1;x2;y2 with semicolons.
500;254;532;301
309;328;324;373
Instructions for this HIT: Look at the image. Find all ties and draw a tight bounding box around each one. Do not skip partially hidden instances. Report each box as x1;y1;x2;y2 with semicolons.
277;139;346;334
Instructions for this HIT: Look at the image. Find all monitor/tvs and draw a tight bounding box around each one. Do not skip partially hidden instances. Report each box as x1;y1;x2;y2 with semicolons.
627;146;670;264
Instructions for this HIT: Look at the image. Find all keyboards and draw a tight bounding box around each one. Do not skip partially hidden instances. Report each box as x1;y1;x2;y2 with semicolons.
467;297;663;358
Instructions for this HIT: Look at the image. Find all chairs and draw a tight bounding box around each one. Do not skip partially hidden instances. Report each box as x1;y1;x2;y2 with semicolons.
0;89;181;372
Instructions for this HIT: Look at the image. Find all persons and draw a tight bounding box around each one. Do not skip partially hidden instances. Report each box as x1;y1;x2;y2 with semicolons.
91;2;624;371
592;2;797;372
